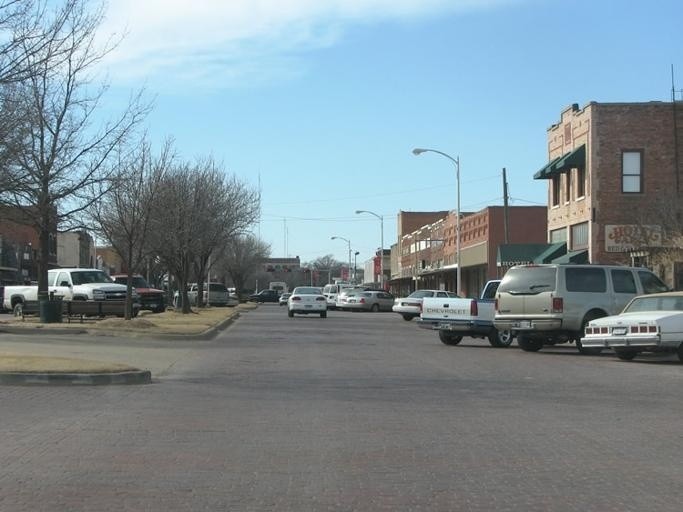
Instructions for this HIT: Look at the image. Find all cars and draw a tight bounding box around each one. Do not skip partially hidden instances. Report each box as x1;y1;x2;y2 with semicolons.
3;268;252;321
323;281;394;311
287;286;327;318
392;288;462;320
580;291;683;362
279;293;292;304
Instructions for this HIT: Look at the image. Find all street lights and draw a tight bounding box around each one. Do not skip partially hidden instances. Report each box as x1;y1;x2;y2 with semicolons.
329;235;352;284
411;147;460;297
354;209;383;290
354;252;360;281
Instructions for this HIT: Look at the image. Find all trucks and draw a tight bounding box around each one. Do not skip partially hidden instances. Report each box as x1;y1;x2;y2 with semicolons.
269;282;288;296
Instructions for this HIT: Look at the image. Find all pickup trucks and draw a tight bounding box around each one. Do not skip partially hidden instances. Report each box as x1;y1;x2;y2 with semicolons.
248;289;281;303
416;279;512;347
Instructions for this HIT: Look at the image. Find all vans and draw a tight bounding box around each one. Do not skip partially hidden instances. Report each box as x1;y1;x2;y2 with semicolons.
491;263;673;355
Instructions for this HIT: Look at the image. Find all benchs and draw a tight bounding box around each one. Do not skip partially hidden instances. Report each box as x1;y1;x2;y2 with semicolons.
22;300;139;322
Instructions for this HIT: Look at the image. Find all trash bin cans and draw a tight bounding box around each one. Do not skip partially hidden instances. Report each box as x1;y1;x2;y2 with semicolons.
40;300;63;323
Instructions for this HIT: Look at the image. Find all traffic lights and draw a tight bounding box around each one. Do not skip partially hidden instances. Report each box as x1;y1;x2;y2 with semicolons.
302;269;309;273
280;268;291;273
266;268;275;272
